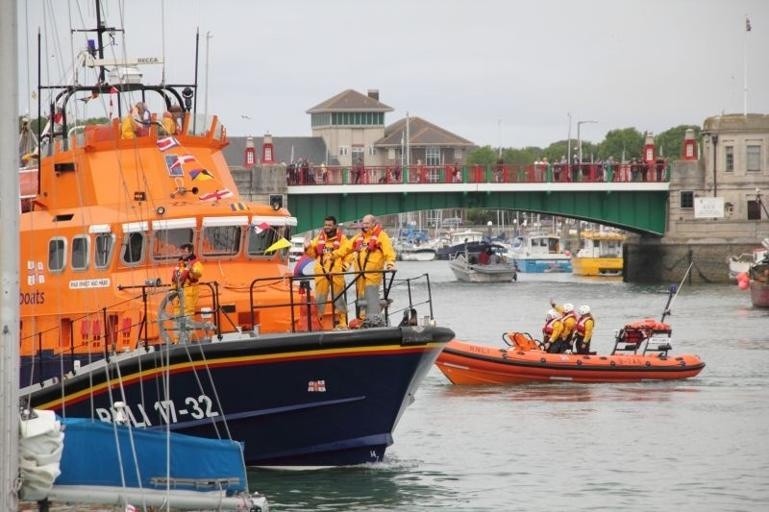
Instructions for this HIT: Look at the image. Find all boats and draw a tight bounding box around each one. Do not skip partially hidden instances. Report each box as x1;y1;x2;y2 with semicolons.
399;230;624;284
19;0;457;468
434;336;708;387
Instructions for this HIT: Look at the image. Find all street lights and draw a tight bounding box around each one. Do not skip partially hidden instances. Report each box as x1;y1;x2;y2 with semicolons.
565;113;597;179
399;109;412;184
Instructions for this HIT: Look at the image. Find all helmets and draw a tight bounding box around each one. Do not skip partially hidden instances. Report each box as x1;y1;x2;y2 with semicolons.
563;304;573;313
578;304;590;315
546;311;558;319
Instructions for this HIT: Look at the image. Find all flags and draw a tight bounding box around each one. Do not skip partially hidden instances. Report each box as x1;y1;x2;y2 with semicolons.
158;136;271;236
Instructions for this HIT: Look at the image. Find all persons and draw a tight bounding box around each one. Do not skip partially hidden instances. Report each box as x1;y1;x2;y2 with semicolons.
321;160;329;184
132;102;151;137
549;297;579;354
166;242;202;345
399;309;418;328
468;157;512;182
522;156;670;182
479;247;497;265
308;216;354;331
163;112;176;135
409;157;463;182
354;155;402;183
328;214;396;327
541;309;564;354
294;240;317;296
576;305;595;355
